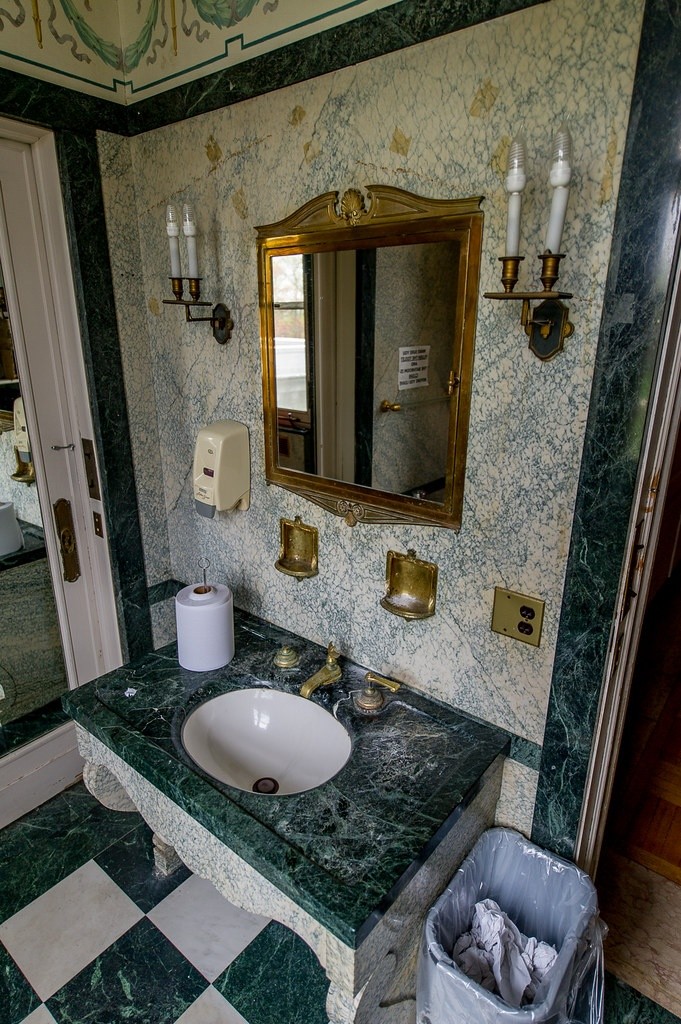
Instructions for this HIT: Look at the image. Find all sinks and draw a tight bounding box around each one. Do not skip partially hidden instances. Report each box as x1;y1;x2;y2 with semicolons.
175;674;356;795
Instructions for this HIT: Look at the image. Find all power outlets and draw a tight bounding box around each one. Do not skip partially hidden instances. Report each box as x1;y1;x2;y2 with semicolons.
491;586;545;647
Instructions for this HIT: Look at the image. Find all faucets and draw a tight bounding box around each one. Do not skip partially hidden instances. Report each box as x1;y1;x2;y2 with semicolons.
300;640;343;699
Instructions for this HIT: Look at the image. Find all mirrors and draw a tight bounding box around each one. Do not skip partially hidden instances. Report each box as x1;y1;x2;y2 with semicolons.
253;184;486;532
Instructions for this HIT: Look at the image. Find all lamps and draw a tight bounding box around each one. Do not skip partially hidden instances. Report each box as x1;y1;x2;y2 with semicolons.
481;125;577;362
166;203;234;344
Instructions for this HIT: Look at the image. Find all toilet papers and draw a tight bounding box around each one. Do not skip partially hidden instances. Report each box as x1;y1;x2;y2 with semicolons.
175;580;235;672
0;501;26;557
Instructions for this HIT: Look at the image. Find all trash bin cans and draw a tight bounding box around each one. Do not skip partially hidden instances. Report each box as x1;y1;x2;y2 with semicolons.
417;827;598;1024
